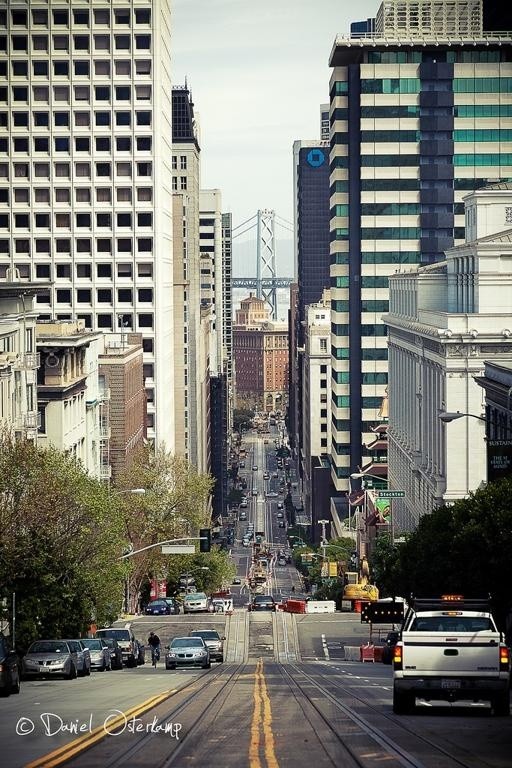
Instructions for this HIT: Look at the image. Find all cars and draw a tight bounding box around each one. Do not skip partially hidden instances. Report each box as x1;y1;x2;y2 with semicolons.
22;625;148;682
163;636;213;670
0;631;20;697
227;411;296;588
142;584;310;615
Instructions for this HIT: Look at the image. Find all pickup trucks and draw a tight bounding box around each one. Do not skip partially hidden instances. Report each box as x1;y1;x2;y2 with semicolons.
385;592;512;719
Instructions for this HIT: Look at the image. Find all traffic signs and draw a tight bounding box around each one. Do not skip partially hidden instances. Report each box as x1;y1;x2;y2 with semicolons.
377;488;406;498
436;410;512;433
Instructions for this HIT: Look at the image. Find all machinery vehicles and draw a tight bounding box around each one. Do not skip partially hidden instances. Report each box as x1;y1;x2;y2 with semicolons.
338;556;381;612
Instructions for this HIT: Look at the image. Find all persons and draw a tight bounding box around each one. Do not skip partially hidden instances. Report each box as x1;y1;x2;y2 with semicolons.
148;631;161;665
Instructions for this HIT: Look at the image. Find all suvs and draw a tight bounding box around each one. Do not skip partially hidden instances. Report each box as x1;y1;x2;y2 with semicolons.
189;629;226;663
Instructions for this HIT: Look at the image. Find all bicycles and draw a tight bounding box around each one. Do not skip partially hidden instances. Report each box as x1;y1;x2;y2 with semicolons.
151;647;159;668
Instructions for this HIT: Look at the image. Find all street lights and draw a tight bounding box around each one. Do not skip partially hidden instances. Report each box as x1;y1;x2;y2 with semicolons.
307;551;331;579
185;566;210;592
115;489;148;497
299;553;318;563
289;535;305;547
347;471;394;545
320;543;354;556
